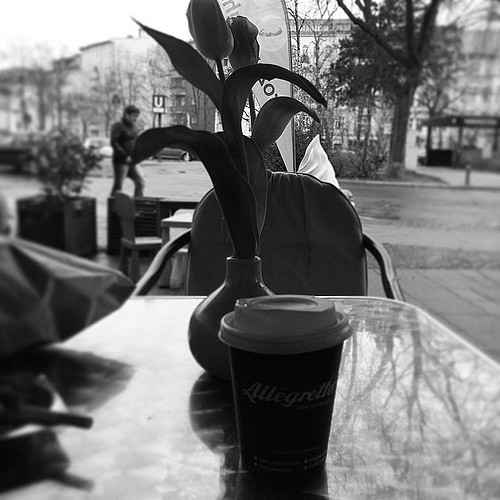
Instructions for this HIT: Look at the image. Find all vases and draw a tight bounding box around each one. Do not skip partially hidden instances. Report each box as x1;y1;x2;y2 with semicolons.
187;255;277;382
107;195;163;256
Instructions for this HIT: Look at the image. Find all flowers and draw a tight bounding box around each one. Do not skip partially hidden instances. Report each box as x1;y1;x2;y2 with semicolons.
126;0;328;262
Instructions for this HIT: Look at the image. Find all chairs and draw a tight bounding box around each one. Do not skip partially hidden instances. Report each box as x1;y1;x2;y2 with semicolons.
114;190;163;283
131;170;405;302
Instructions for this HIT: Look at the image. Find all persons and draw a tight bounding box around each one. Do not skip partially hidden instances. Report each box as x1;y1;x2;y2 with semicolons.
109;105;145;198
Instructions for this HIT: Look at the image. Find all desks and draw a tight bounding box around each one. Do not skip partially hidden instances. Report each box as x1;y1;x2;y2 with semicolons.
157;195;204;285
0;296;500;499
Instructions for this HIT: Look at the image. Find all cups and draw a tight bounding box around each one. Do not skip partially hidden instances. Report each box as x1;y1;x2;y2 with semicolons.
218;295;354;482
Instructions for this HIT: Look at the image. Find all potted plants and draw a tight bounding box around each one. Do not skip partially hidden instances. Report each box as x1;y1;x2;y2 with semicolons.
16;123;105;256
